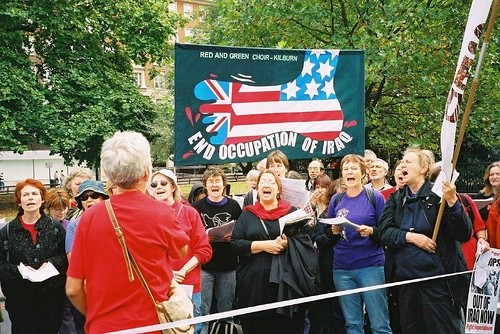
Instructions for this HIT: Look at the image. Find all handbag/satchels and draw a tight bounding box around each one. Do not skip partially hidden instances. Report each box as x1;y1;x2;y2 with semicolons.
154;279;196;334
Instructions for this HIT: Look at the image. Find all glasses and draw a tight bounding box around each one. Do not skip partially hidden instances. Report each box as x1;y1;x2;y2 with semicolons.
80;192;101;201
150;181;171;188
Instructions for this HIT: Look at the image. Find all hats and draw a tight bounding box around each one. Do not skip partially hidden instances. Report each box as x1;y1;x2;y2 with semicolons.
74;179;109;210
147;169;180;198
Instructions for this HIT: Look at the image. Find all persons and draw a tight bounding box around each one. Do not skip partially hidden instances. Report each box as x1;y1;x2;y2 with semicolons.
65;130;191;334
0;143;500;334
